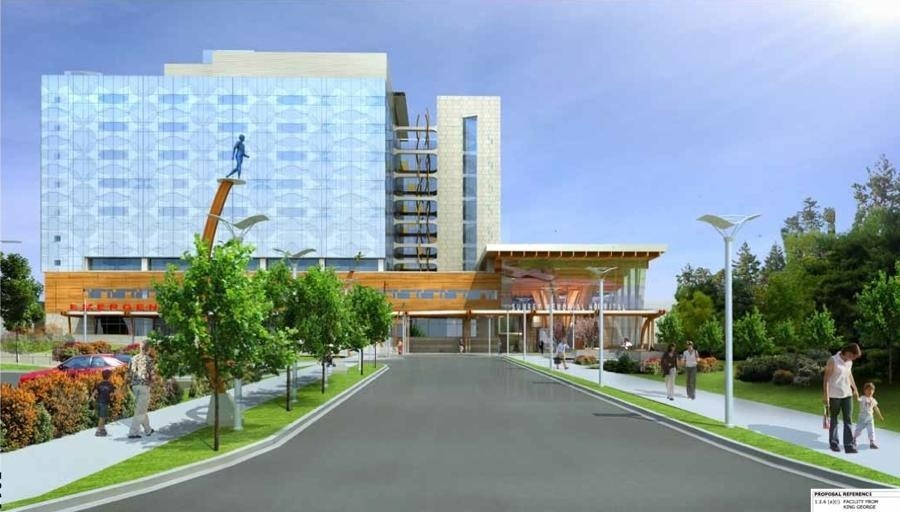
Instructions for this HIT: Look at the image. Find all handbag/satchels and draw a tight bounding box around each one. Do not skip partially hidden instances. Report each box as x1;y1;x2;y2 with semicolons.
553;357;562;365
822;404;832;429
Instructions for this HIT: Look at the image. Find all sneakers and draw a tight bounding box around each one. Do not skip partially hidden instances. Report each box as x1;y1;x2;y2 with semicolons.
869;439;880;449
687;396;696;400
853;437;857;445
128;434;142;438
829;443;840;452
666;396;674;401
145;428;154;437
844;445;858;454
95;429;108;436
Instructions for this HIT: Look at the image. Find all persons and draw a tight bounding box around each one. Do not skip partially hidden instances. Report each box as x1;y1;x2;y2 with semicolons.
226;135;250;179
661;342;678;400
822;343;858;453
556;338;570;369
89;369;116;437
539;340;544;353
682;341;700;400
621;338;633;350
458;338;464;353
396;336;403;355
852;382;884;449
127;339;157;438
